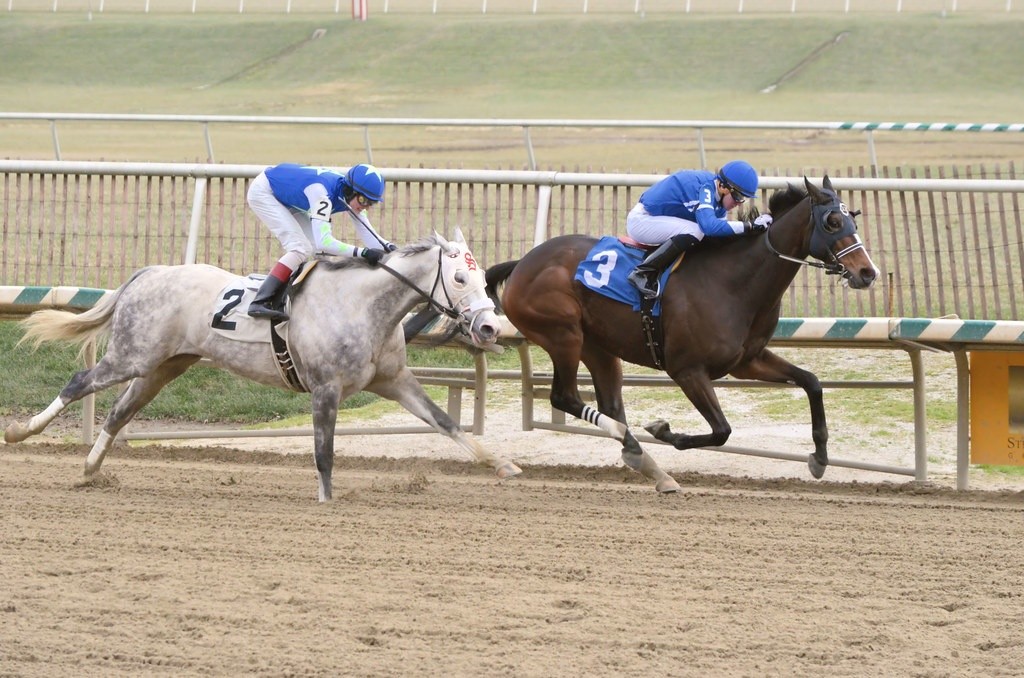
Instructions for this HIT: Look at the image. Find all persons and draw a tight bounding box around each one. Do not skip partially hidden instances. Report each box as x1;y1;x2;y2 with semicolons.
248;164;398;319
626;161;773;296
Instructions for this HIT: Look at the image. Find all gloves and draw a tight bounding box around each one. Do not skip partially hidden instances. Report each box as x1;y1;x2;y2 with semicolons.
361;247;385;266
751;214;773;231
389;245;397;252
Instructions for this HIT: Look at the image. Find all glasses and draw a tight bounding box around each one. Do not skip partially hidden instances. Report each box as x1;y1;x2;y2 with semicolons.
348;168;374;206
719;169;745;203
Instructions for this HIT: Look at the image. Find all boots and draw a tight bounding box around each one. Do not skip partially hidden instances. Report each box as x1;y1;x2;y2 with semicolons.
248;273;289;321
627;237;681;296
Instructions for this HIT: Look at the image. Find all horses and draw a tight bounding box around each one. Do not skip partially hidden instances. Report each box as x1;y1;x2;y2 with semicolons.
404;175;880;493
4;228;522;504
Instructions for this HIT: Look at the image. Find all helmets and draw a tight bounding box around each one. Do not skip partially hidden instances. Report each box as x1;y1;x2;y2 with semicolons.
345;163;386;202
716;160;758;198
